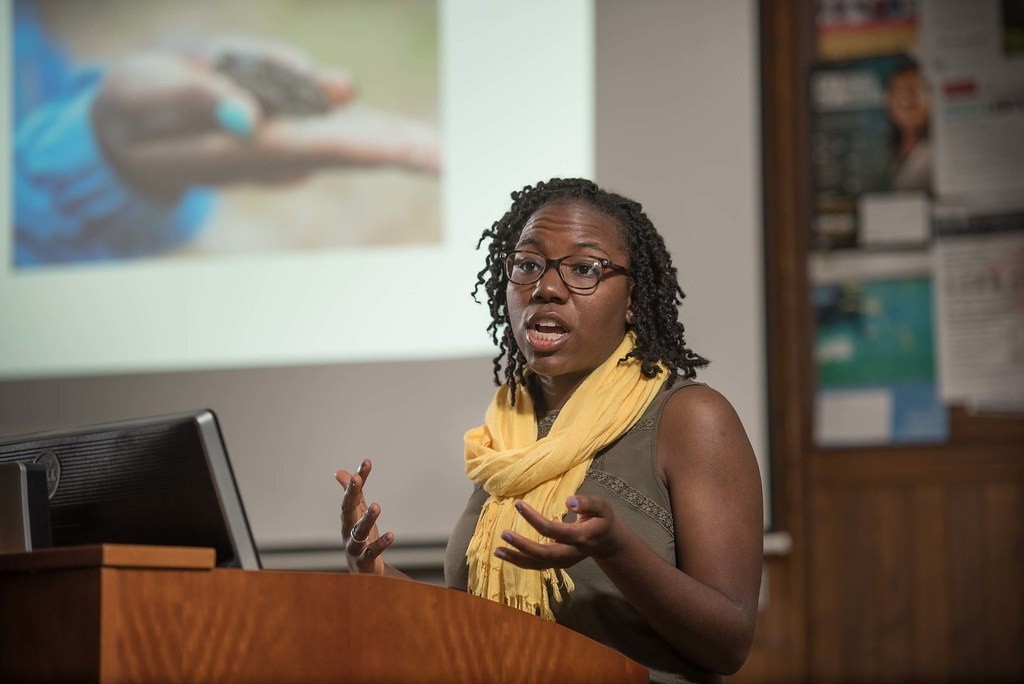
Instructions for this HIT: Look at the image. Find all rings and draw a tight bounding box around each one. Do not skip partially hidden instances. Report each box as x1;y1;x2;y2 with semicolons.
351;531;369;543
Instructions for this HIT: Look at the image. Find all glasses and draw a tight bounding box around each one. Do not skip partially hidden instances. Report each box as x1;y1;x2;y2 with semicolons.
499;250;630;290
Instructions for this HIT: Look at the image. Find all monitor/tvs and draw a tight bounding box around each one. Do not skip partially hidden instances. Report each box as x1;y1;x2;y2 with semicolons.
0;408;264;570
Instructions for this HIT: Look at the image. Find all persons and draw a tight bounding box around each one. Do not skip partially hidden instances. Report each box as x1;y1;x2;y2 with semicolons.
334;177;764;684
879;66;932;190
9;0;437;268
